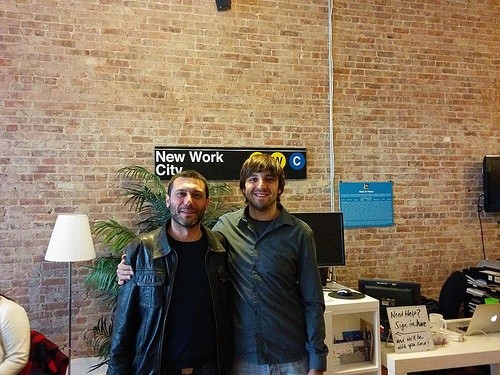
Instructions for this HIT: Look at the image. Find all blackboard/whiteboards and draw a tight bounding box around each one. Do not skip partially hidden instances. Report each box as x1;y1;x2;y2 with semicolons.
387;306;435;353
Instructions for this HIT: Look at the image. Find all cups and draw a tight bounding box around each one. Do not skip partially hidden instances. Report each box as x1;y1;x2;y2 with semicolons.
484;298;498;304
429;313;447;330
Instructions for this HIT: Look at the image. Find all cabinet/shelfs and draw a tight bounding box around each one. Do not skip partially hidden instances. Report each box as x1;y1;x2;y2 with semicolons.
323;278;382;375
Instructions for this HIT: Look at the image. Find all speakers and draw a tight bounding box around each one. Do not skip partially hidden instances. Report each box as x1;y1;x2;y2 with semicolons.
215;0;232;11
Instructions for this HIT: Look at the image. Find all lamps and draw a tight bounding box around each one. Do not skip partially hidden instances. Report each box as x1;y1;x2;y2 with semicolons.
44;214;97;375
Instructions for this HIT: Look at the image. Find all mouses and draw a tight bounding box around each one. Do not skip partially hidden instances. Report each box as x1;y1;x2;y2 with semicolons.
337;289;352;296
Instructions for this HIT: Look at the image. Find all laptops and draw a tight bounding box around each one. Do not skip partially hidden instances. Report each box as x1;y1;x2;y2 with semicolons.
443;303;500;336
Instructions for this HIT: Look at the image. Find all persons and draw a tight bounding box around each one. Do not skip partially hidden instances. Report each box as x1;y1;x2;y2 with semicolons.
0;294;30;375
105;171;225;375
117;154;329;375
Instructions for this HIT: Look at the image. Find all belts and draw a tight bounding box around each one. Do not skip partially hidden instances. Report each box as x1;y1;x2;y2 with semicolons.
165;364;202;375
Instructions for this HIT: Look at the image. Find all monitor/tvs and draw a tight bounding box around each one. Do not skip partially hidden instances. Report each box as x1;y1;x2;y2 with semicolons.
291;212;346;292
482;155;500;212
358;278;420;344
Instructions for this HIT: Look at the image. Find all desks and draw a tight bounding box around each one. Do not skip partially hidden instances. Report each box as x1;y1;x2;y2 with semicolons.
382;318;500;375
